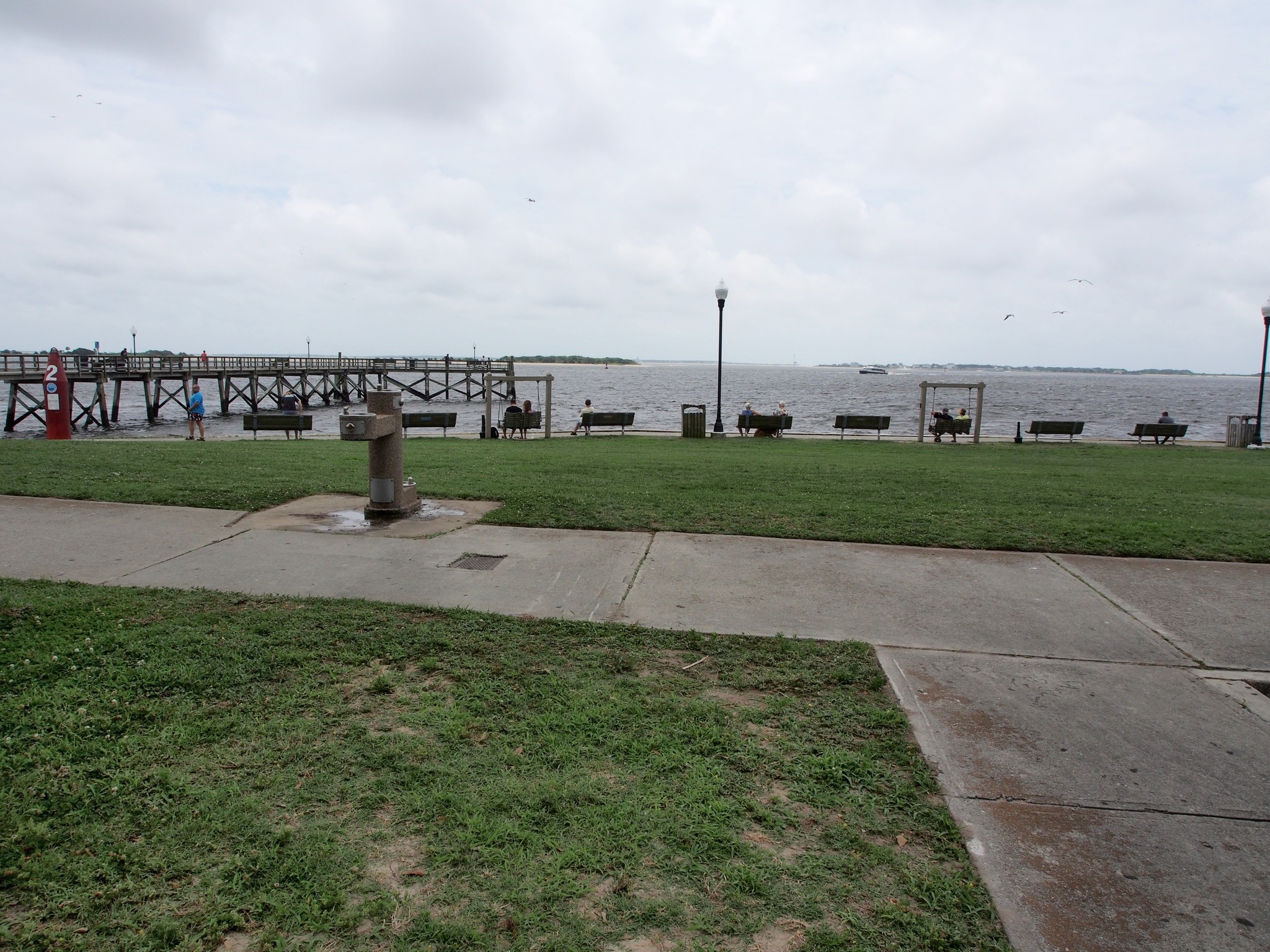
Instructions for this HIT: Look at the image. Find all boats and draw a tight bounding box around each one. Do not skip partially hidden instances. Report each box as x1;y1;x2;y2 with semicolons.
859;363;889;374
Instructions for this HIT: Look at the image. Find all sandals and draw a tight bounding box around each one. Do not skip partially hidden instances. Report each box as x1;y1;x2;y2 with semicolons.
185;436;195;440
585;433;588;435
570;432;577;435
197;437;205;441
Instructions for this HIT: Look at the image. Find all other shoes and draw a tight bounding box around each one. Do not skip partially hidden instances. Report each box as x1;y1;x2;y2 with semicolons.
1160;442;1164;444
951;440;956;442
934;438;941;442
1155;442;1159;444
777;435;781;437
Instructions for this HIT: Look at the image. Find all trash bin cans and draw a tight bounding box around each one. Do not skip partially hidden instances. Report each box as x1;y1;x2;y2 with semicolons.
681;404;706;438
1227;415;1257;447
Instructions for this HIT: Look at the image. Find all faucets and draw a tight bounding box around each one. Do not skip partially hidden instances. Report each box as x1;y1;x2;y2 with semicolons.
343;406;350;415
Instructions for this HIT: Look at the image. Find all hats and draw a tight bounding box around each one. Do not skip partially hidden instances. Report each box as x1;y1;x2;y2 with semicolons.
779;401;785;405
943;408;949;414
957;408;966;413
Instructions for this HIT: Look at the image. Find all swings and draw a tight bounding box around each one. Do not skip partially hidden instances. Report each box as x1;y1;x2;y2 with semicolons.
497;381;543;429
927;386;972;435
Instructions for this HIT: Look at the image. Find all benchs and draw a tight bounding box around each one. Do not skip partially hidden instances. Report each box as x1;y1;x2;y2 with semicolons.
242;413;313;440
401;411;458;437
1025;420;1085;442
498;411;543;431
735;413;794;438
833;414;892;441
1128;422;1192;446
578;411;636;435
929;418;974;436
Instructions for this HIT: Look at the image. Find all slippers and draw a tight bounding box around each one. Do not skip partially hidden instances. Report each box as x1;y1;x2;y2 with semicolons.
509;434;512;438
501;436;507;439
514;436;519;438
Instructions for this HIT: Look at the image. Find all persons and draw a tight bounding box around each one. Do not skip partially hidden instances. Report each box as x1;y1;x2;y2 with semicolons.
501;399;522;439
1153;411;1174;445
951;408;970;442
183;383;207;441
571;399;595;436
930;407;953;442
200;350;207;367
737;402;761;436
772;400;788;438
281;392;303;440
518;400;536;439
120;348;128;360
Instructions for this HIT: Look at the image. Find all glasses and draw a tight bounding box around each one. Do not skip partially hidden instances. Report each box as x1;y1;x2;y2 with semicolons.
751;406;751;407
192;387;198;389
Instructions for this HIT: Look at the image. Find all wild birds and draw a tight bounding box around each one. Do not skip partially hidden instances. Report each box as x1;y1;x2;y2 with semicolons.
1067;279;1093;285
1003;314;1014;321
525;198;535;202
1051;311;1069;314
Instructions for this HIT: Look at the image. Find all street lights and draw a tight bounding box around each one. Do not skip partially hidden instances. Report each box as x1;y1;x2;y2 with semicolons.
473;343;476;367
306;336;311;366
130;326;137;368
714;277;729;433
1253;298;1270;445
